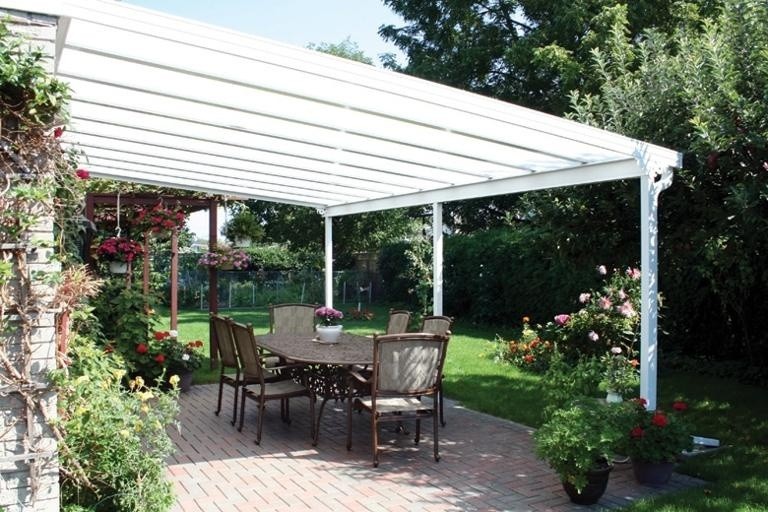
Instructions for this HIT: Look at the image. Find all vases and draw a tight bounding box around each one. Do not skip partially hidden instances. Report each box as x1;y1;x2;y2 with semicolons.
168;368;193;393
629;451;688;489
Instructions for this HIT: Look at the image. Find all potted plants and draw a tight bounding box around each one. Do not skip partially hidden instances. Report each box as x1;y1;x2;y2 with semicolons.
531;402;637;506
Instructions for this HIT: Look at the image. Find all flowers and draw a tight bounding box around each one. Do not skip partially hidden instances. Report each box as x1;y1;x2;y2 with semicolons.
154;354;166;362
170;329;179;339
631;396;647;405
651;413;667;425
181;353;190;361
672;401;687;411
629;426;645;440
163;330;170;338
187;341;194;349
135;343;148;353
194;340;204;348
154;330;163;339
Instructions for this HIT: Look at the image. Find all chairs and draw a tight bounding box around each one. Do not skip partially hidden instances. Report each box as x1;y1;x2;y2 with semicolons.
353;331;451;468
385;310;412;334
420;312;454;426
208;311;287;427
229;317;318;445
268;303;317;333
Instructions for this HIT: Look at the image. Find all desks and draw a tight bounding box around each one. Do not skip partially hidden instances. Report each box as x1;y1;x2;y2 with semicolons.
252;333;404;450
